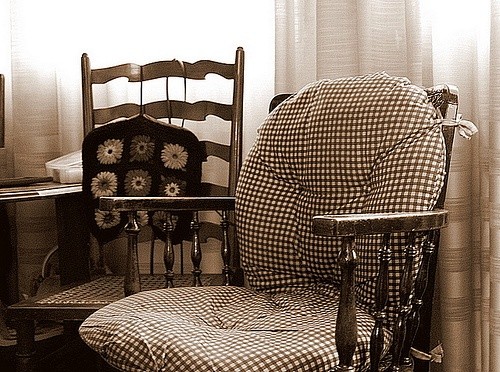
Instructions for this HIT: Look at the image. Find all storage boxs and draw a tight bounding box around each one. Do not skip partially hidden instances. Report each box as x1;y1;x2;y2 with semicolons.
44;150;84;184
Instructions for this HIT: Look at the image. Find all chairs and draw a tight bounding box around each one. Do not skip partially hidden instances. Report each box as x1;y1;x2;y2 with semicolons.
75;73;461;372
5;46;245;372
